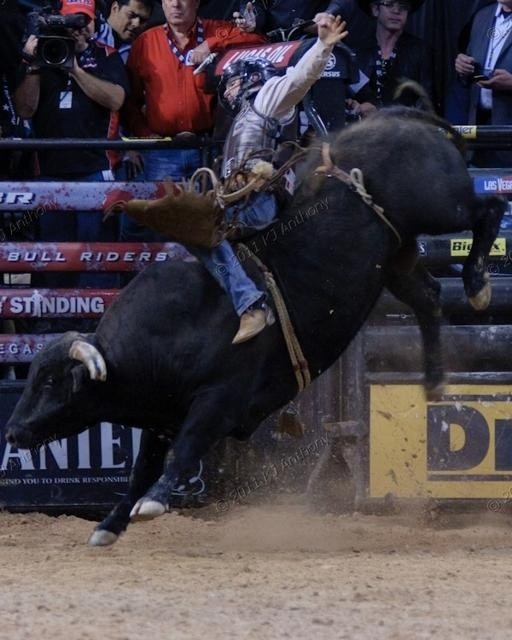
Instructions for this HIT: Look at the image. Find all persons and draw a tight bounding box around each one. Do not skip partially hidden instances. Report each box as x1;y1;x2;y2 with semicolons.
14;0;512;344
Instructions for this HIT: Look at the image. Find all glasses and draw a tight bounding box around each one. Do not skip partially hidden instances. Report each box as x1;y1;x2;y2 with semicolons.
377;1;409;10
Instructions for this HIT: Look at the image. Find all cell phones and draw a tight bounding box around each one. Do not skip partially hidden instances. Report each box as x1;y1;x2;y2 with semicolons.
474;75;489;82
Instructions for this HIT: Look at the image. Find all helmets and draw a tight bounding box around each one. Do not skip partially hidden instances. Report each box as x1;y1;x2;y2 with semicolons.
219;56;276;86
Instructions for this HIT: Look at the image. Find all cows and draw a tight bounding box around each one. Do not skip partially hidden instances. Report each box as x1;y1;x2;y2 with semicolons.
4;80;509;547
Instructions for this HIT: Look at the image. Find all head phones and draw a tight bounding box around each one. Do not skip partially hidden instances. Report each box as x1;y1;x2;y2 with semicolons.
51;0;106;34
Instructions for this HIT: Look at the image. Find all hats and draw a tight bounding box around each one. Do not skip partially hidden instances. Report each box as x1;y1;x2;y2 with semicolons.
59;0;97;18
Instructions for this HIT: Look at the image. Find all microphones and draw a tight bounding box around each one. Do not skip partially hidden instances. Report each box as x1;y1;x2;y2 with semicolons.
86;24;106;44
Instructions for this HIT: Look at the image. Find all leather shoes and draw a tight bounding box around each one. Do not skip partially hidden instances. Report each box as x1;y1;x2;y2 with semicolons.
231;304;275;345
